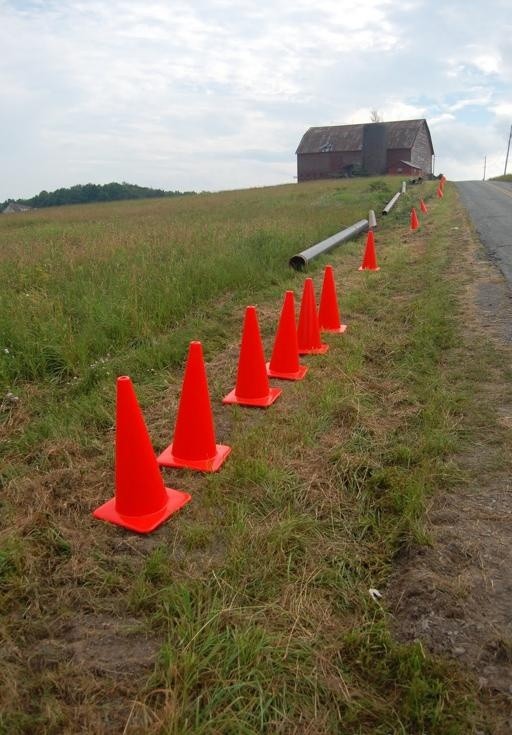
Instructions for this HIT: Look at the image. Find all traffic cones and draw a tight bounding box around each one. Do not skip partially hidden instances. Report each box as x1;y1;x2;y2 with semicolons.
436;174;449;199
357;229;383;271
410;208;420;231
418;196;430;214
